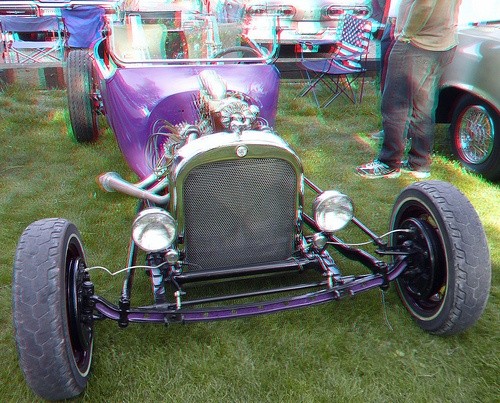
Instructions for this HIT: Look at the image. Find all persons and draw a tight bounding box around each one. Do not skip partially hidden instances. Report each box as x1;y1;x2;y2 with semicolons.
371;1;413;140
354;1;462;179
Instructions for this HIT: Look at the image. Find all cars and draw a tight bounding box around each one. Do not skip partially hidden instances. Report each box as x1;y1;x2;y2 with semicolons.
13;3;492;402
434;26;500;179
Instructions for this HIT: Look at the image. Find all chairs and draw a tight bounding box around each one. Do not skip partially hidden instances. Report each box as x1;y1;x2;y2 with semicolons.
180;20;245;64
111;15;168;65
295;13;372;110
60;4;109;61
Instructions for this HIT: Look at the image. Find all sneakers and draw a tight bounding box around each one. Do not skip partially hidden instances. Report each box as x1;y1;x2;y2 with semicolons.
371;129;384;139
353;158;402;180
400;159;432;179
